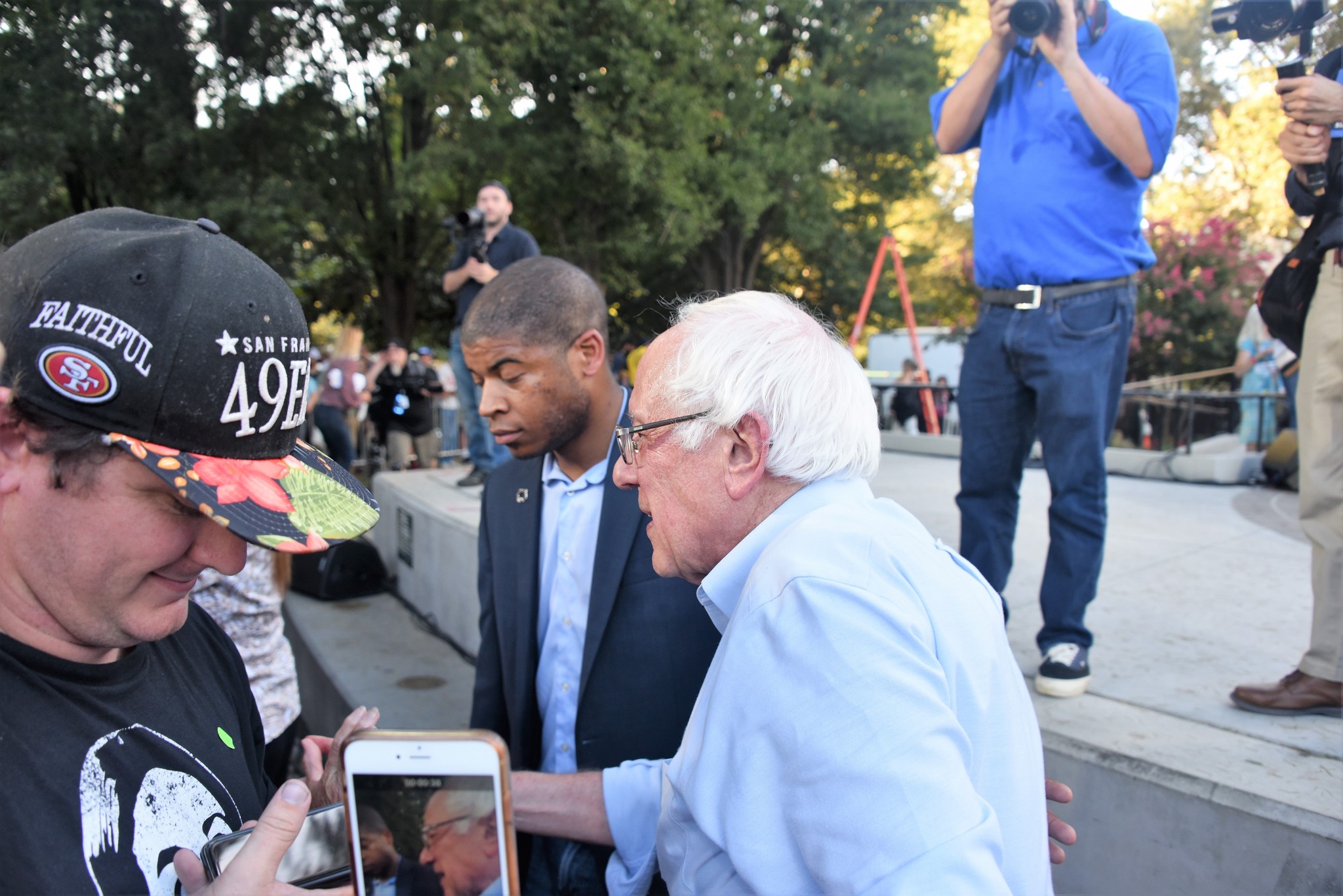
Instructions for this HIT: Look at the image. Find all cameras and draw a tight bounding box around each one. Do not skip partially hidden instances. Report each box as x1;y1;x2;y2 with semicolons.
457;207;486;263
1211;0;1330;43
1009;0;1060;38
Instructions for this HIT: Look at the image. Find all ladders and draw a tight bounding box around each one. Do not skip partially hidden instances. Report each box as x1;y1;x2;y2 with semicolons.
848;233;940;436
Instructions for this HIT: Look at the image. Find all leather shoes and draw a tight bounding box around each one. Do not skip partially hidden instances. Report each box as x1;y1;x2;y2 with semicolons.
1228;669;1343;717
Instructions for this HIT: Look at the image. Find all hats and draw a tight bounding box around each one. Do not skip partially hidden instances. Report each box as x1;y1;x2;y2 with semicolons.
0;206;382;554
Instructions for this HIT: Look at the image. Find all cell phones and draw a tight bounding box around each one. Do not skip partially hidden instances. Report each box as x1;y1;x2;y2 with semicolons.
339;730;521;896
201;800;351;891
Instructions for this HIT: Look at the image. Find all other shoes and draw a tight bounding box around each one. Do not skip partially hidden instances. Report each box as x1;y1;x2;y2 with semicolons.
1035;642;1092;698
457;466;490;486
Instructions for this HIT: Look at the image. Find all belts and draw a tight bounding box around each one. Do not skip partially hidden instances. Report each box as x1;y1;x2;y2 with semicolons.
980;276;1130;311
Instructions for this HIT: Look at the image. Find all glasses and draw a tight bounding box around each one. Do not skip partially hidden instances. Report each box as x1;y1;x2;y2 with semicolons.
612;409;716;466
418;813;474;847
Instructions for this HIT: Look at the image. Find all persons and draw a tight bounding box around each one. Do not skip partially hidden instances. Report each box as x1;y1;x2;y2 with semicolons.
0;206;382;896
1230;48;1343;718
460;257;1077;896
929;0;1179;697
300;326;461;472
189;543;309;788
440;182;538;487
890;358;954;432
356;774;503;896
505;294;1056;895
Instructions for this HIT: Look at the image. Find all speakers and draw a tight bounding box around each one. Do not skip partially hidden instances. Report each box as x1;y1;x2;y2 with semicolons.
289;536;389;601
